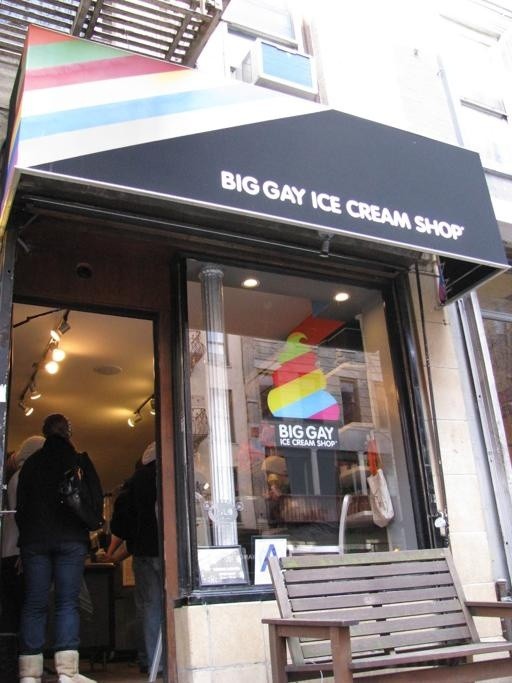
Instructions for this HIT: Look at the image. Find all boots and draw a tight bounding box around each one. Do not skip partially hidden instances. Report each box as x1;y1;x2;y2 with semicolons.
18;653;44;682
54;650;97;683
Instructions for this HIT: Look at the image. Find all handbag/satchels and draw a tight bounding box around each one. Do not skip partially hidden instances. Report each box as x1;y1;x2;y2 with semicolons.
367;440;396;529
56;453;106;532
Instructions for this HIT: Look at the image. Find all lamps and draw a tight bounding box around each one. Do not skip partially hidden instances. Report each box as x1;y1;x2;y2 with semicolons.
318;232;334;259
128;393;155;427
13;309;71;417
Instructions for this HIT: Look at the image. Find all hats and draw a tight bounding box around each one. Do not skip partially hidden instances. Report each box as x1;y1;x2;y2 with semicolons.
261;455;288;477
142;440;157;466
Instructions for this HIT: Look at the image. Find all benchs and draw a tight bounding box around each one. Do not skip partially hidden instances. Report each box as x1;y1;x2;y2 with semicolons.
260;547;512;682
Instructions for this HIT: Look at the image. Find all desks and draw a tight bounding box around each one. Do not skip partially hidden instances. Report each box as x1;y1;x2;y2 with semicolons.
44;561;139;672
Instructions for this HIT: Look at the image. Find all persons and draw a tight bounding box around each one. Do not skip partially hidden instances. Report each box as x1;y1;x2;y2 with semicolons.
261;454;293;535
95;442;162;679
14;413;104;683
2;437;46;683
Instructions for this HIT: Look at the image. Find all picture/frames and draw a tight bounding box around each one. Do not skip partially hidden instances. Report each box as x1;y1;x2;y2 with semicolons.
197;545;250;588
250;534;290;587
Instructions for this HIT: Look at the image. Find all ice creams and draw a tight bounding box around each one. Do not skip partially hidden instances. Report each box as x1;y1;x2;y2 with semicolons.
267;332;344;495
